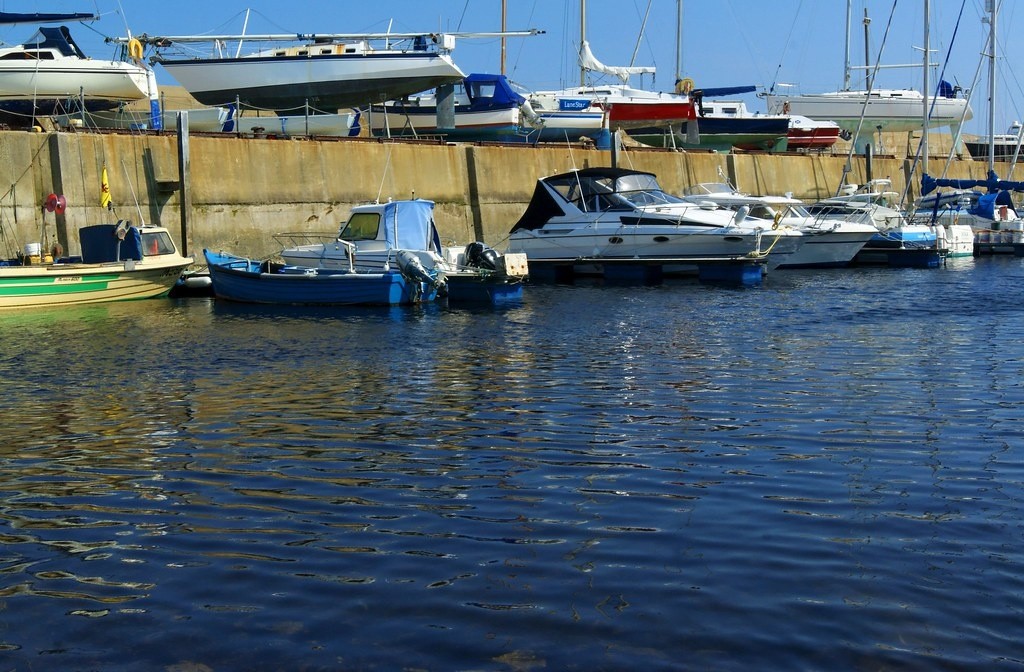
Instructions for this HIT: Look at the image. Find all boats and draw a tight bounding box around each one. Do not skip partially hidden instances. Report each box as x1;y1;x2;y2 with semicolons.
201;189;530;312
0;1;1024;276
0;157;194;311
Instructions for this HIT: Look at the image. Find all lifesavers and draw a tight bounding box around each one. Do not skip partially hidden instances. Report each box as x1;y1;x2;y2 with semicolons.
129;38;143;59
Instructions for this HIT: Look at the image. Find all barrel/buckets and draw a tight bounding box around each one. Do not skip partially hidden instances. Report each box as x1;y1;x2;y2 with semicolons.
25;243;40;265
978;221;1024;243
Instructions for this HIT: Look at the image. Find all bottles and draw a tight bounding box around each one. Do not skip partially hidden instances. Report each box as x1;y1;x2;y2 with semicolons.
32;126;42;132
